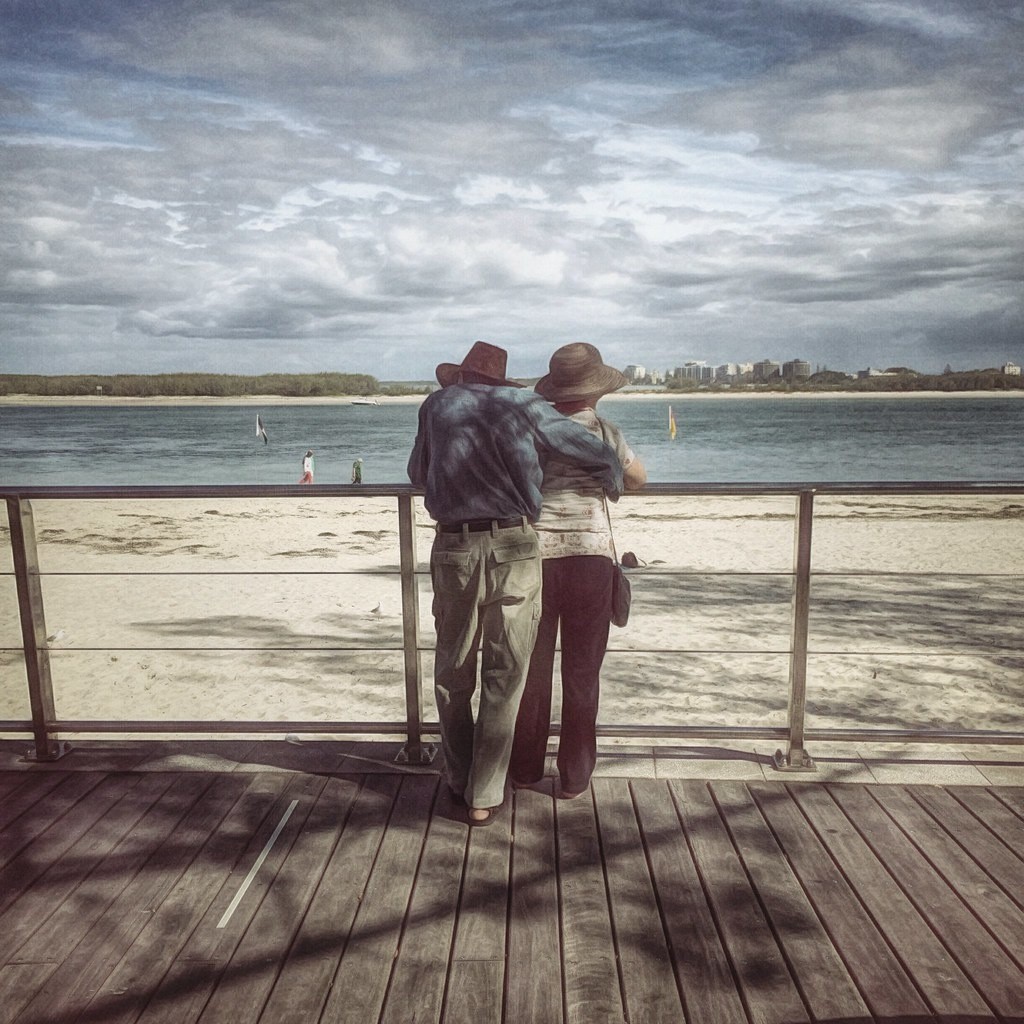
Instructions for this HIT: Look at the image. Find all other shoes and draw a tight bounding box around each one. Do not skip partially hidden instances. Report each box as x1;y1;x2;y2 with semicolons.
560;780;591;799
507;767;544;788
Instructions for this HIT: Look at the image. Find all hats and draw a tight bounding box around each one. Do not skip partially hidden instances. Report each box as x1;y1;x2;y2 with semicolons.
534;343;626;403
436;341;527;389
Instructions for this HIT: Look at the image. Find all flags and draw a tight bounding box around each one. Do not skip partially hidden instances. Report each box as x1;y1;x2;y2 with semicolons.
669;406;677;440
257;414;268;444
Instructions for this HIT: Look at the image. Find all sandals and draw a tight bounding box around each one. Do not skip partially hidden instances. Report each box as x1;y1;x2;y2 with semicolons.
448;783;464;805
464;801;506;826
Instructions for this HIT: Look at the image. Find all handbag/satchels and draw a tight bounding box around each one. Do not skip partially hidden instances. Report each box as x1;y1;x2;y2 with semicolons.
610;566;631;627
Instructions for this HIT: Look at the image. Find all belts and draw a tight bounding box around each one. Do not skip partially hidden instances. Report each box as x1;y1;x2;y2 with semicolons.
439;518;529;533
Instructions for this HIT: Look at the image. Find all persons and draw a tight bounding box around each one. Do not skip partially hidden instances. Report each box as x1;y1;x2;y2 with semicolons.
508;343;646;799
407;341;624;827
298;450;314;484
352;458;363;484
